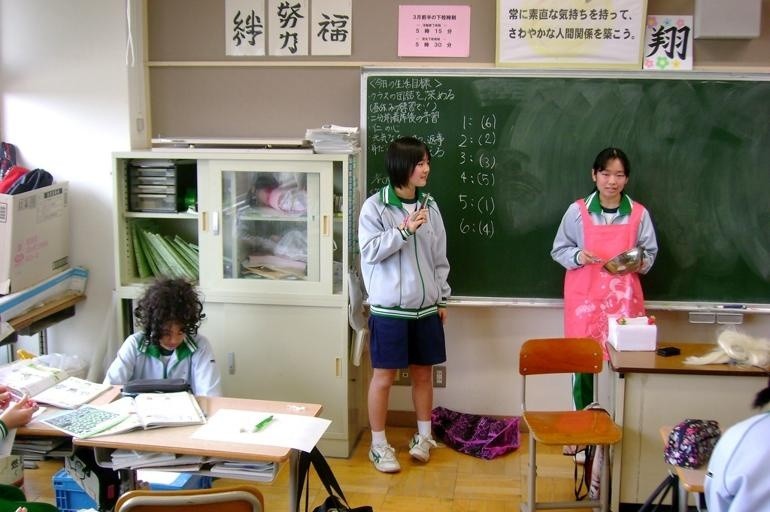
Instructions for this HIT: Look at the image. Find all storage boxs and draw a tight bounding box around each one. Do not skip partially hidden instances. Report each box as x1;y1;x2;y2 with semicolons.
0;179;73;295
51;467;99;512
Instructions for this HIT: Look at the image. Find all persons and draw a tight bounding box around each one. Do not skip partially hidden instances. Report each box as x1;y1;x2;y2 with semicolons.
356;136;452;472
701;370;770;511
0;385;62;512
549;148;661;464
103;278;222;397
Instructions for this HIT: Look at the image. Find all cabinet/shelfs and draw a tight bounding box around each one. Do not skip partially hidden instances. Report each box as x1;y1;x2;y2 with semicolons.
111;147;363;307
113;294;367;458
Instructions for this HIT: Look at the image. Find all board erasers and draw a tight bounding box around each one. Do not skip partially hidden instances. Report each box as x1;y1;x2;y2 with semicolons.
688;311;715;324
717;312;744;325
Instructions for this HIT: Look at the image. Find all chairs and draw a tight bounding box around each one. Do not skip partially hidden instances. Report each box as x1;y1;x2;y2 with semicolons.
113;487;265;512
517;339;624;511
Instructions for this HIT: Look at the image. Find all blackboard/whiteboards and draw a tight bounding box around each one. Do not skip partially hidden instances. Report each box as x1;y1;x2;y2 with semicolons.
359;65;770;315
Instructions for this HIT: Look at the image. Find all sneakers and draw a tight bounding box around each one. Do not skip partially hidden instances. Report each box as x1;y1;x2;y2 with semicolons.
408;431;437;463
368;442;400;473
574;449;586;464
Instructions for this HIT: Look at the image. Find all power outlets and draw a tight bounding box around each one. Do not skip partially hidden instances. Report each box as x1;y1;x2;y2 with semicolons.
433;365;447;388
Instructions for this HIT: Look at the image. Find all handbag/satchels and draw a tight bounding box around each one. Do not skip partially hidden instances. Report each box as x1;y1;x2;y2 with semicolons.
664;419;721;469
431;406;520;460
313;496;373;512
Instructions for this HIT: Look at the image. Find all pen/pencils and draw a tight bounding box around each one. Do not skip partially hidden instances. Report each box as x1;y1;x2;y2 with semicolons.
713;305;743;309
7;392;41;410
251;414;274;433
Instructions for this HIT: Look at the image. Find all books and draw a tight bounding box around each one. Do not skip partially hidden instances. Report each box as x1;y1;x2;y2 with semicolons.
131;222;200;287
0;357;278;482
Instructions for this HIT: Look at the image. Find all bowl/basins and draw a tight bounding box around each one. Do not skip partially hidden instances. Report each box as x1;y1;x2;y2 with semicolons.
600;245;643;277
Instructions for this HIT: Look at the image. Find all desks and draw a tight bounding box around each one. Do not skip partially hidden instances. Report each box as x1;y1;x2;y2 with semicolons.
71;395;323;512
660;424;724;512
603;341;770;512
0;381;129;512
5;292;87;358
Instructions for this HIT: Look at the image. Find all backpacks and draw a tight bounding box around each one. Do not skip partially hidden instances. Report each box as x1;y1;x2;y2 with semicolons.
0;166;29;194
574;401;611;500
5;168;53;195
1;142;16;178
